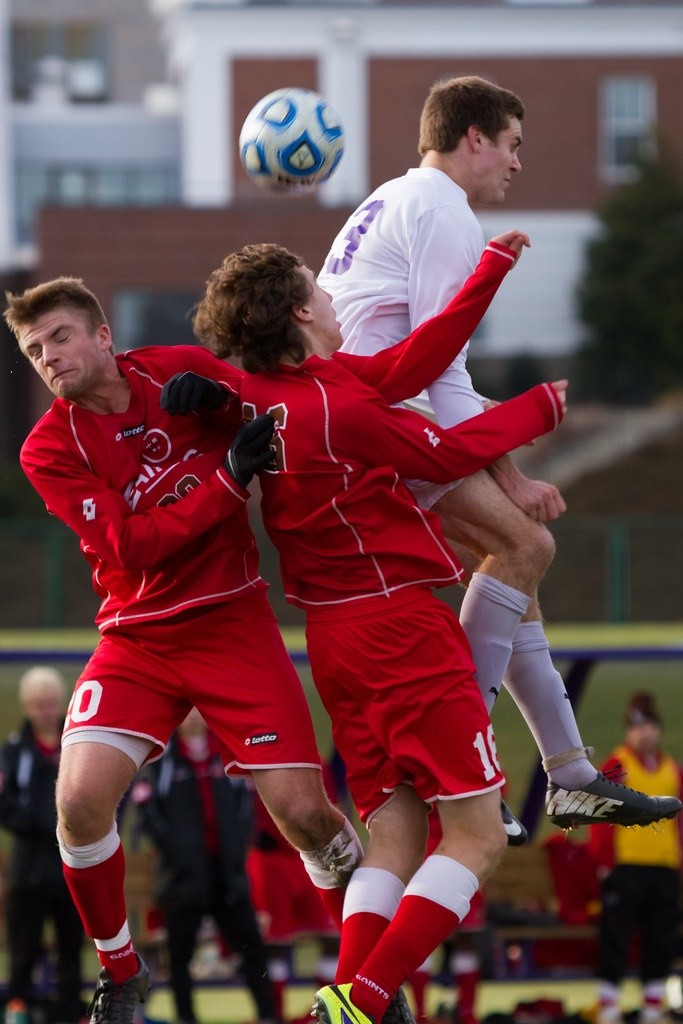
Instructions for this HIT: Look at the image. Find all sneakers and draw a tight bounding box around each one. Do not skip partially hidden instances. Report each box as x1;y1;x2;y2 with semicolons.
501;799;529;847
544;765;682;829
311;982;372;1024
86;952;150;1024
382;986;416;1024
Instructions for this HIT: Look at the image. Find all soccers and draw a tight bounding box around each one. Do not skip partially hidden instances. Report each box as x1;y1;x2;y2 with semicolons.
237;84;344;195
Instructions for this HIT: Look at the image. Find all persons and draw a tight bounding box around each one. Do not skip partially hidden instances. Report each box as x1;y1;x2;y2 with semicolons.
589;693;683;1023
3;277;366;1024
190;226;573;1023
0;663;337;1024
315;75;683;847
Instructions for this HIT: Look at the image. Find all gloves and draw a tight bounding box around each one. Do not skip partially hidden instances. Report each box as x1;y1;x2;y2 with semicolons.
221;413;276;491
158;371;225;418
